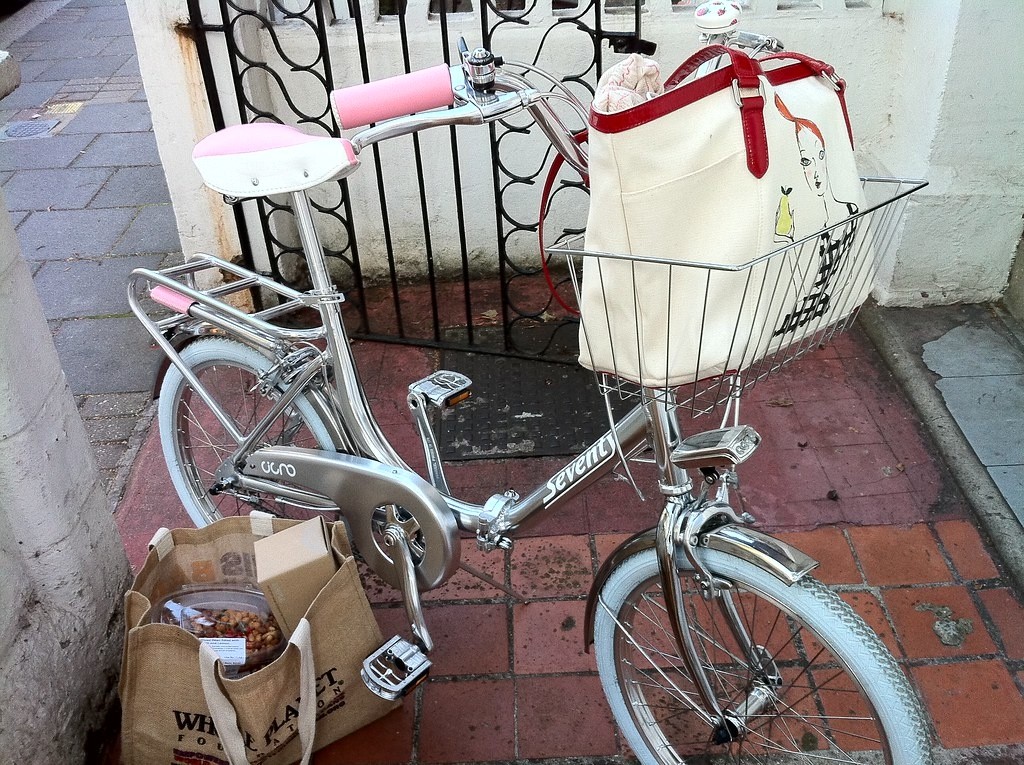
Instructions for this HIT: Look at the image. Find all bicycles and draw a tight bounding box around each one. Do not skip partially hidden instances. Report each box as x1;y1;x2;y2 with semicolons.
125;2;935;765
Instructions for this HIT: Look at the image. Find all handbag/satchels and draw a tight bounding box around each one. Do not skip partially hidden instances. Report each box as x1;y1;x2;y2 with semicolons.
116;500;403;764
578;41;870;373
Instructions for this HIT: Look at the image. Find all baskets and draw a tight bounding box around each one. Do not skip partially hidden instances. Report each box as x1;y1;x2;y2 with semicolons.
545;173;930;420
